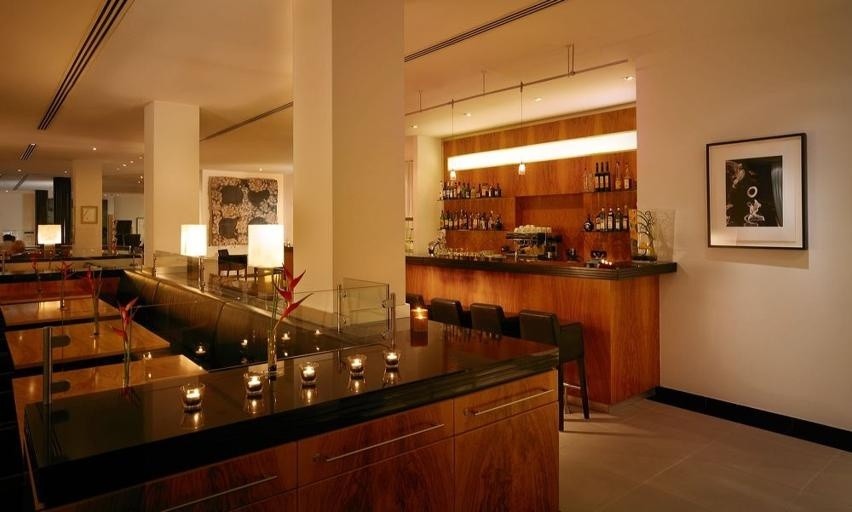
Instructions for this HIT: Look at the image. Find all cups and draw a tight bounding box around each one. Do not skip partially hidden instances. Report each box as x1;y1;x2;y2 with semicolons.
512;224;553;234
180;369;399;431
179;345;402;412
435;246;493;258
409;308;429;333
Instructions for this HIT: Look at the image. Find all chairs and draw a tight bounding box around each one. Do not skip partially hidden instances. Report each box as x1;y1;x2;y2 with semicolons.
406;292;590;432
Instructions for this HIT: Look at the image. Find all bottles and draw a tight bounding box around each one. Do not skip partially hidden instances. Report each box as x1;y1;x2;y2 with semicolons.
439;208;502;230
438;179;502;200
583;161;633;192
583;205;629;233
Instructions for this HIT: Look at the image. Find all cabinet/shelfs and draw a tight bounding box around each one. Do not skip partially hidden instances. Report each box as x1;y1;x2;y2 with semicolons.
579;188;636;233
435;197;512;233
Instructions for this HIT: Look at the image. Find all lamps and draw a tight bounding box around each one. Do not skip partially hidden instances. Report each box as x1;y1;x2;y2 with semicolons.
178;223;208;286
37;224;62;256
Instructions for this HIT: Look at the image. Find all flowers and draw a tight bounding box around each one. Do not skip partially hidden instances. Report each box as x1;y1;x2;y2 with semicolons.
259;266;316;369
55;260;75;303
30;256;46;293
82;264;103;328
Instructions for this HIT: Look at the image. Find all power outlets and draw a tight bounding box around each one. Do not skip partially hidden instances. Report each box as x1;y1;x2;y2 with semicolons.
246;223;285;304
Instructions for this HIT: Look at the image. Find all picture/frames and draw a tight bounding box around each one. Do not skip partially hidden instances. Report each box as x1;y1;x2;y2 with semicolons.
79;205;99;225
199;169;285;249
706;133;809;250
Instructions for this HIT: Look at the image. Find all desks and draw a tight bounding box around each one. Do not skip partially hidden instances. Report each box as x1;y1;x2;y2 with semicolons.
0;281;210;459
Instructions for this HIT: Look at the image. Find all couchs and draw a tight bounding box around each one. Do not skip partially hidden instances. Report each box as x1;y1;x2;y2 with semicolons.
217;249;246;281
